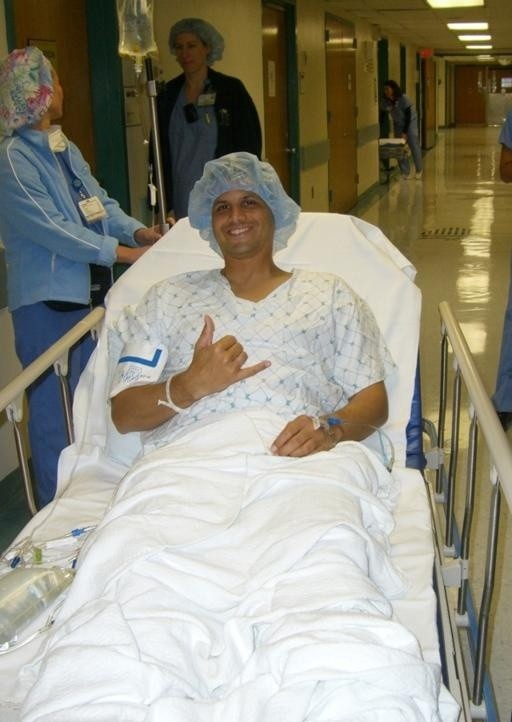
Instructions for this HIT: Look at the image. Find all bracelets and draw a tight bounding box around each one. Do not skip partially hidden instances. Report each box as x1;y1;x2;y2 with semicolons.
157;368;193;414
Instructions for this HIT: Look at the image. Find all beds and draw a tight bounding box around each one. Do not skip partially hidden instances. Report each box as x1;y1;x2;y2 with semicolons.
0;300;512;722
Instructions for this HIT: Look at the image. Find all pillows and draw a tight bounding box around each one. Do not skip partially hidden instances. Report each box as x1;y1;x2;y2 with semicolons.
96;329;396;469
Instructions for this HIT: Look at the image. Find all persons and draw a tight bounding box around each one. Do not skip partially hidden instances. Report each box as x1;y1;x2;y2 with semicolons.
379;80;423;179
1;49;174;506
146;18;261;234
489;112;512;431
24;151;435;722
